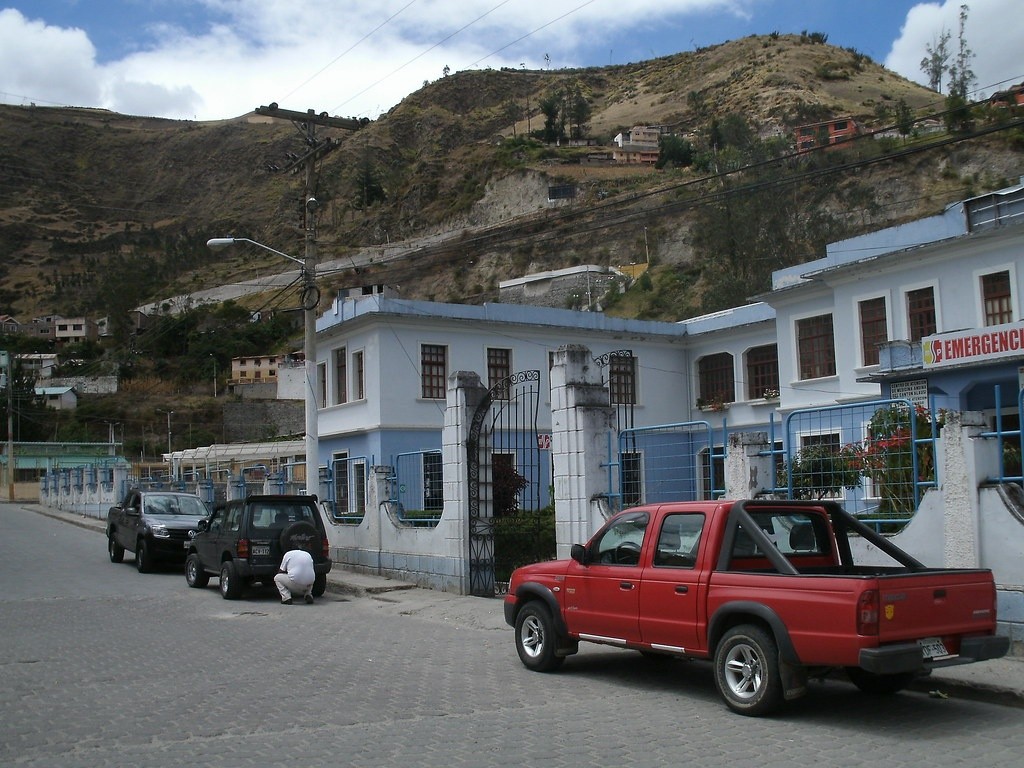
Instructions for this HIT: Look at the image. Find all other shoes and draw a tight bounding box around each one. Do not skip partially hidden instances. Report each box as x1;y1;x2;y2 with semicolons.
281;599;293;605
305;595;313;603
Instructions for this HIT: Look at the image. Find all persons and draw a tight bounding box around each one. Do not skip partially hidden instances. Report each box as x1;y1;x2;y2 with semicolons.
274;540;317;604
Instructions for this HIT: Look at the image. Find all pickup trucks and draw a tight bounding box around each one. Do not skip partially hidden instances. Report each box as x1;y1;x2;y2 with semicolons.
503;499;1011;719
105;487;212;574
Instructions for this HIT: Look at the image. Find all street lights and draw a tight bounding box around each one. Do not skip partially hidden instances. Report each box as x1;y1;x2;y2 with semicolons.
156;408;176;483
207;236;320;494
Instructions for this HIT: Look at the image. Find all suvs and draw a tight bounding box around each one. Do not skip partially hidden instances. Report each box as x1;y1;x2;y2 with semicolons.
184;494;334;602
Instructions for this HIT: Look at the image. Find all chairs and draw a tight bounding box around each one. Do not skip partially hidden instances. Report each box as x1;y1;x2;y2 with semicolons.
789;523;815;554
268;513;290;528
733;528;757;558
658;524;682;556
237;515;241;525
